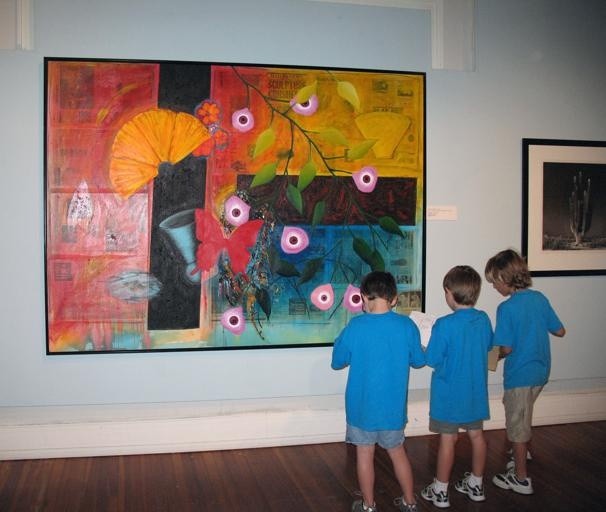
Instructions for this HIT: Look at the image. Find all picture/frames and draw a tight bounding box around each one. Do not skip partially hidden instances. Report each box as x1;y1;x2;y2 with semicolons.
520;137;606;277
43;55;428;355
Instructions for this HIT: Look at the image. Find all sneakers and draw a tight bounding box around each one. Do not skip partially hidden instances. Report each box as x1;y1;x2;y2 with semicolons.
454;474;486;502
491;470;534;495
350;497;377;512
421;482;451;509
506;447;533;470
392;494;419;512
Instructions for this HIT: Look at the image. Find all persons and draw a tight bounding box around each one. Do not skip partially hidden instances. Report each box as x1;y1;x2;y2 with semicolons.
484;245;567;497
330;269;427;511
422;264;497;508
